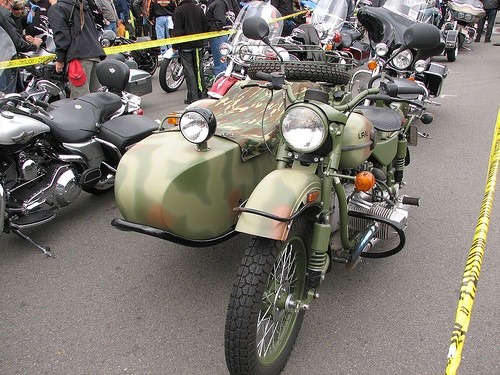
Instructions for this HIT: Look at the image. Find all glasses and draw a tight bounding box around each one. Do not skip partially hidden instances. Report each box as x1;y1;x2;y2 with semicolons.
12;6;25;11
6;0;14;8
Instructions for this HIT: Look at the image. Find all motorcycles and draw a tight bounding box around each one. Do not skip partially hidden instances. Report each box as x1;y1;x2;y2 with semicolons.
100;36;158;78
348;1;450;139
0;77;60;114
110;16;447;375
310;0;370;67
206;2;325;100
0;59;164;256
443;0;486;62
159;47;215;92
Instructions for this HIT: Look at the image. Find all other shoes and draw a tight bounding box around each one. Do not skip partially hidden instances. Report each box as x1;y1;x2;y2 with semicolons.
184;99;196;104
474;37;480;42
484;39;491;42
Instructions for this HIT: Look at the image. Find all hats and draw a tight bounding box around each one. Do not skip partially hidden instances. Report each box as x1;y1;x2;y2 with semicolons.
69;59;86;87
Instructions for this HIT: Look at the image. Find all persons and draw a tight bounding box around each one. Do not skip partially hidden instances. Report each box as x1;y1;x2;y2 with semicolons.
474;0;500;43
0;0;50;94
172;0;211;105
88;0;179;60
206;0;314;77
48;0;106;100
